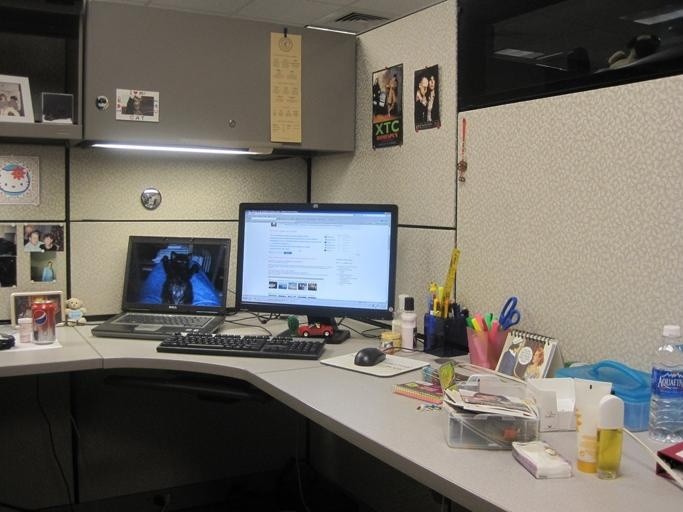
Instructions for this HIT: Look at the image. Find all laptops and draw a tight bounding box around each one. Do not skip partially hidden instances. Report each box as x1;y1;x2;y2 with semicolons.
92;235;231;339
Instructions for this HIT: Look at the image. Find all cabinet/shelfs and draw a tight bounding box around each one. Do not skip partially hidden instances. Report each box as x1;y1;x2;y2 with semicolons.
77;3;357;158
0;0;88;147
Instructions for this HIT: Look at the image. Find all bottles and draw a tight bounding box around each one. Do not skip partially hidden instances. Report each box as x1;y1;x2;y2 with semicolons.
649;325;683;444
381;295;418;353
18;318;32;342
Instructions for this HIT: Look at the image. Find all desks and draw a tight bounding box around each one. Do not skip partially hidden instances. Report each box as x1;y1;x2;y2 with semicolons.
0;322;683;512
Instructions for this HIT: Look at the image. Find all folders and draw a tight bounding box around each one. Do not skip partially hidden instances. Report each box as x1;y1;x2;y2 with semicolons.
656;439;683;485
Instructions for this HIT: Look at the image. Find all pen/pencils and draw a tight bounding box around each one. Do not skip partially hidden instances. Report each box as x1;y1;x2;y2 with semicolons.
466;312;507;367
424;281;461;323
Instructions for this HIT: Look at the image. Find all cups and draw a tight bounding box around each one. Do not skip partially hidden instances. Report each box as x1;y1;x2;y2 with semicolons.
424;314;451;358
466;328;511;370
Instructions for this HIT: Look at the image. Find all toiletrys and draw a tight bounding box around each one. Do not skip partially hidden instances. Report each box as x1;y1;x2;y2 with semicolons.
574;377;612;472
596;395;625;480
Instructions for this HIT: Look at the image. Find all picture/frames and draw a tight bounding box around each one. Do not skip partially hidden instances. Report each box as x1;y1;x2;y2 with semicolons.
12;291;64;330
0;75;35;123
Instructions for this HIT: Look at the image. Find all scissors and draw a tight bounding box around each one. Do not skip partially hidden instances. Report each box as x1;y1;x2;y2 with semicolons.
499;297;520;332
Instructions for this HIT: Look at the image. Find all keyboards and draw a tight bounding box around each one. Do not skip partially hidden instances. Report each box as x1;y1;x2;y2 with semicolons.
157;329;324;361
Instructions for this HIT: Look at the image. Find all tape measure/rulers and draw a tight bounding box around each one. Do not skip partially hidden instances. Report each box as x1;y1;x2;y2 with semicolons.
444;248;460;298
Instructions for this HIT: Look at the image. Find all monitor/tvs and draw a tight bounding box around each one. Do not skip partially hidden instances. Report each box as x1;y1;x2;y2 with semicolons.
235;203;400;344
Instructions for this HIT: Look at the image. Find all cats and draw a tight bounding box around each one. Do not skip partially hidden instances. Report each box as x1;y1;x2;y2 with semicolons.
160;251;200;305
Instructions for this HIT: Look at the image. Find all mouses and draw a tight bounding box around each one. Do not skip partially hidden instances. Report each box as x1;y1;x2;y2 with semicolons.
354;347;386;366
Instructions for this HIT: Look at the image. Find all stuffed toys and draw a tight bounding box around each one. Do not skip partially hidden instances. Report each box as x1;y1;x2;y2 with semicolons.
64;297;86;327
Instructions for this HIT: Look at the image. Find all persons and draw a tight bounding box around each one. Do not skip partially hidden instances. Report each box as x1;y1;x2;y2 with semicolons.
18;303;31;319
126;96;143;114
523;346;544;380
0;94;19;116
373;76;397;118
24;225;57;251
497;337;523;376
415;74;438;123
40;261;54;282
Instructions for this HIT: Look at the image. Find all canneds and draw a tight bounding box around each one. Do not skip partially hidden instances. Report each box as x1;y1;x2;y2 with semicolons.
31;299;56;345
